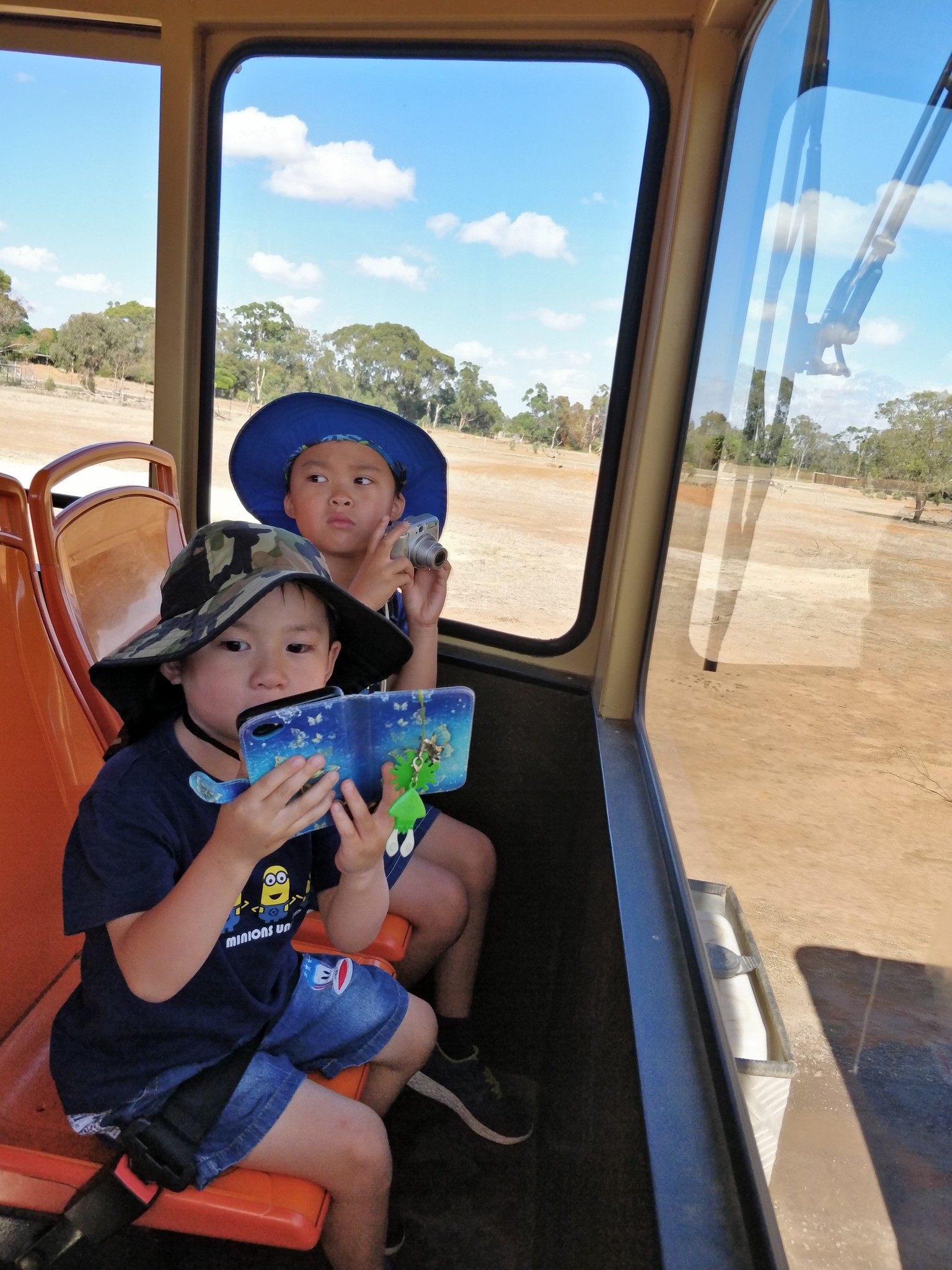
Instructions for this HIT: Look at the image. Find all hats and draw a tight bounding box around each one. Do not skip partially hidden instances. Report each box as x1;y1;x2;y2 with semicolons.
89;520;416;762
229;391;448;539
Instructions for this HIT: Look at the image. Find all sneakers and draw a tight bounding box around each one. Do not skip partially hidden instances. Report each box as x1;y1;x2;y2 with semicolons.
404;1041;534;1145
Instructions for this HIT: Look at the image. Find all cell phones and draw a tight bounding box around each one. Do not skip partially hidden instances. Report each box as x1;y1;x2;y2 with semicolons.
236;686;345;732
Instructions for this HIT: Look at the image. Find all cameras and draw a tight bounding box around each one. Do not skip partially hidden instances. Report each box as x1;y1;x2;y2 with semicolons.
383;514;448;571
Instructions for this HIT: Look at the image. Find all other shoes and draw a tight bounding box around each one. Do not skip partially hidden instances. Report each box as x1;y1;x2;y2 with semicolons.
383;1222;405;1254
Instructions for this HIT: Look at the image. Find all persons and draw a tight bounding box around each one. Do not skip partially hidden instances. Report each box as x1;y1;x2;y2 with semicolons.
49;520;437;1270
228;392;533;1145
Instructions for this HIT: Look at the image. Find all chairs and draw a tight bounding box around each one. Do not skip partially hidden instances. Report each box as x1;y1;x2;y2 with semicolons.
0;441;416;1270
